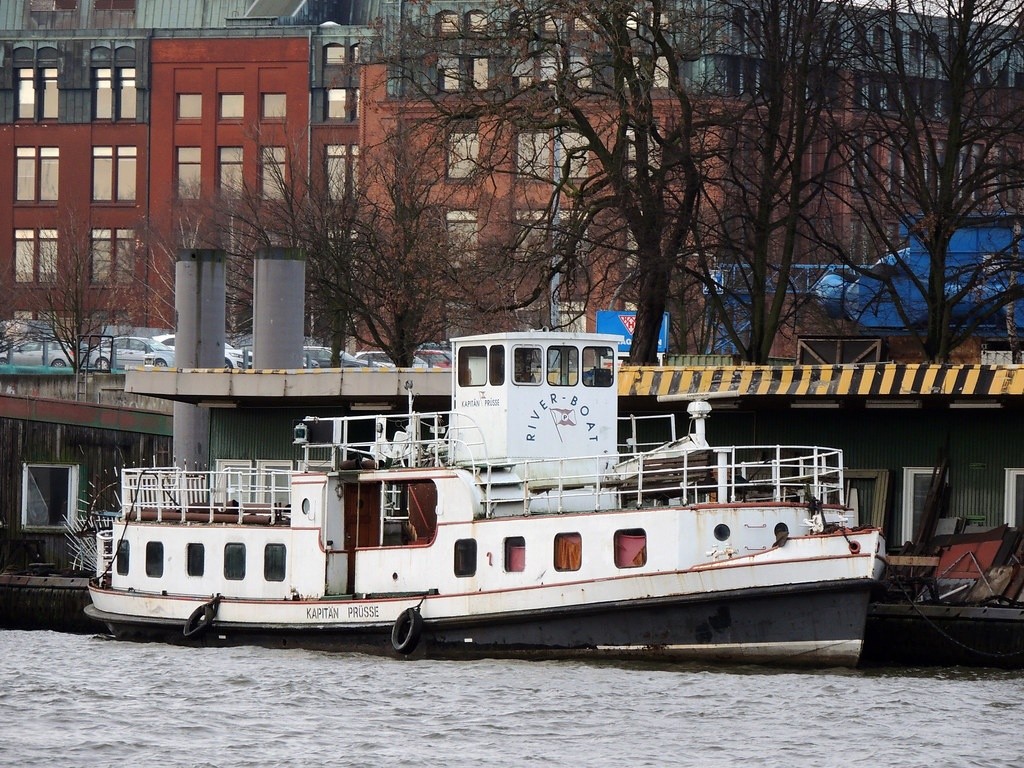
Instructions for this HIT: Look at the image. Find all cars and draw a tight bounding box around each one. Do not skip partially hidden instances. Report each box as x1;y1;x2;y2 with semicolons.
2;337;82;369
354;352;436;370
93;336;177;372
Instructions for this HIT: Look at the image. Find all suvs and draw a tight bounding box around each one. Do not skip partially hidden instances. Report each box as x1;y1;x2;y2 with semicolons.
304;346;379;369
156;335;250;370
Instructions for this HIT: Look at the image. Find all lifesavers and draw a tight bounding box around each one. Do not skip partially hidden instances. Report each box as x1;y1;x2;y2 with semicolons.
184;605;215;641
393;609;420;652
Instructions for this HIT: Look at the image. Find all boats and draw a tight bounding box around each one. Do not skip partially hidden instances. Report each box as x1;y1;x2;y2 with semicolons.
82;328;885;666
